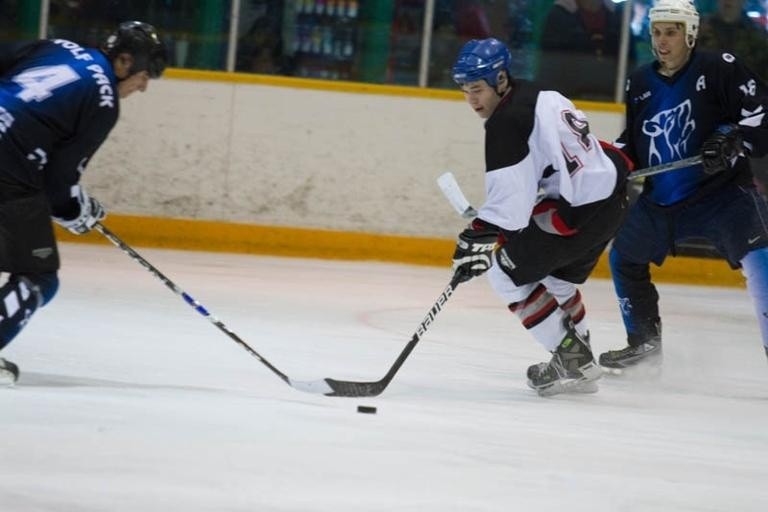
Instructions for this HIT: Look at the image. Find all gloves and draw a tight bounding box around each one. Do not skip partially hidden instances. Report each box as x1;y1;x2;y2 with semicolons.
702;123;747;175
55;184;106;235
453;229;499;281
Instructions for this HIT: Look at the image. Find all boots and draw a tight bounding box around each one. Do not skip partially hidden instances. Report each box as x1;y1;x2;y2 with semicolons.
528;314;661;389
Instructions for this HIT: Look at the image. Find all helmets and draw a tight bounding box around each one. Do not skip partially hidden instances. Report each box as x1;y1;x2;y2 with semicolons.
452;37;512;87
648;0;700;48
102;21;165;79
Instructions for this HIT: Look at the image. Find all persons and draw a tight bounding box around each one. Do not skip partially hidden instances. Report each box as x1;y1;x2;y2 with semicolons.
1;19;167;381
219;17;296;75
597;0;768;368
450;1;767;104
450;35;632;389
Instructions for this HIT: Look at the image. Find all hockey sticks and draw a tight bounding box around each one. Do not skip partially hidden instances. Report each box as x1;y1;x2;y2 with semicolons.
323;279;459;397
93;222;340;394
437;156;703;218
